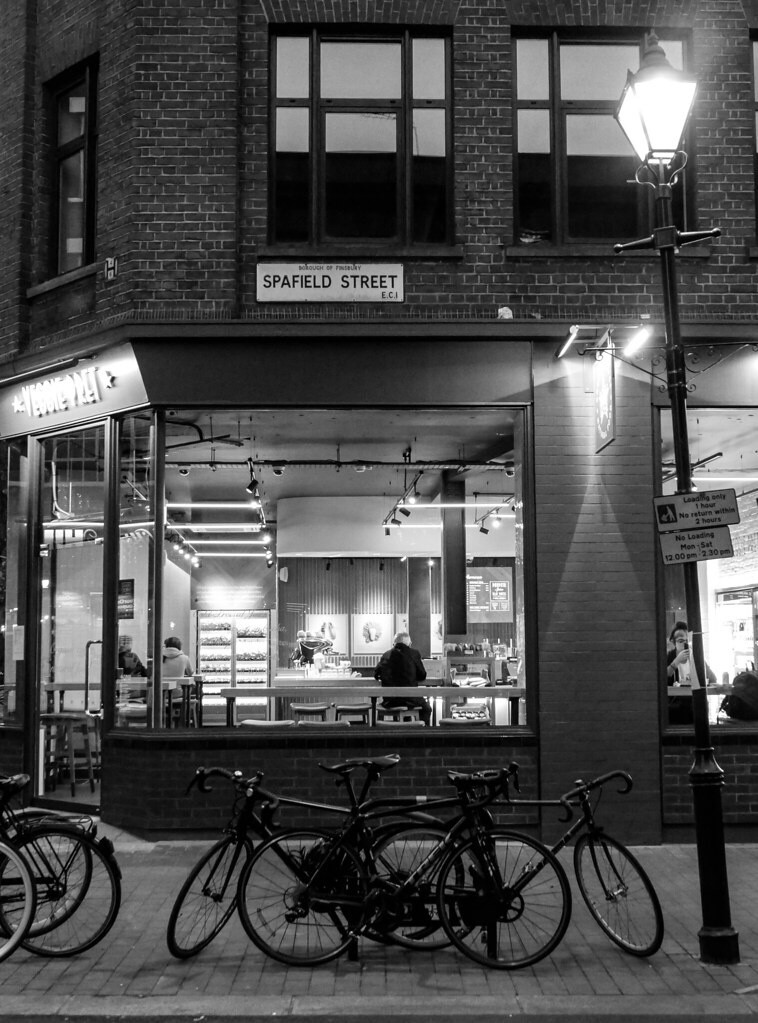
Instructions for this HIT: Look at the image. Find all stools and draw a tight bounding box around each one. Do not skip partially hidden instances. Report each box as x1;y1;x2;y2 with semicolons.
375;705;421;723
290;701;329;723
331;702;372;723
39;714;95;797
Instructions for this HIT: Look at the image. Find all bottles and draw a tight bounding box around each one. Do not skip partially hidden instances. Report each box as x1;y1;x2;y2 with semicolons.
445;637;493;657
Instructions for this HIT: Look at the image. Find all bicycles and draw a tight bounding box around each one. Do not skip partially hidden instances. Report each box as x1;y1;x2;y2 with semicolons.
0;768;122;965
166;754;664;970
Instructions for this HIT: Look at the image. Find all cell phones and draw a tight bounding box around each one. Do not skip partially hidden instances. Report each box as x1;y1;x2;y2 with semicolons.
683;643;689;651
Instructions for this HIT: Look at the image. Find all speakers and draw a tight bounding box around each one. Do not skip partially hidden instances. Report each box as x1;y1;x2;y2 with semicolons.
279;567;288;583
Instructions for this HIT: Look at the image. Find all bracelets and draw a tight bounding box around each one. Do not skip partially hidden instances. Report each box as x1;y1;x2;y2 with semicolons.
670;663;677;672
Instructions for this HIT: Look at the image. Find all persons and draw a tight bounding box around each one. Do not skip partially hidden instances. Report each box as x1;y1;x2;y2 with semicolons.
374;632;432;725
666;622;717;726
116;636;148;699
161;636;194;699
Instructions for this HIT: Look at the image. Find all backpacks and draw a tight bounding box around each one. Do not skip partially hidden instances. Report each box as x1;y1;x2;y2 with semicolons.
719;672;758;721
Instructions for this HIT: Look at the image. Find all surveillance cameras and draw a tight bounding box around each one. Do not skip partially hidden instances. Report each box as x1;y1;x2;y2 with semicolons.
272;466;285;477
504;468;516;477
177;464;192;476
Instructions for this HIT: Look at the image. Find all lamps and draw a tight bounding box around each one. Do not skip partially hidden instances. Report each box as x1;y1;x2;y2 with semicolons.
391;513;402;526
554;324;578;358
479;521;489;535
382;494;479;529
399;507;411;516
245;480;259;494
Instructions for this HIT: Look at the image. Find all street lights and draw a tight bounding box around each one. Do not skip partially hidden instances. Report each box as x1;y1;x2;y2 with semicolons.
612;26;740;966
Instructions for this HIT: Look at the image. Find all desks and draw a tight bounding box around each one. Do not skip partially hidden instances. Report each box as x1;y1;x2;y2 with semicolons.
0;681;177;730
220;686;527;727
272;676;443;726
122;677;195;729
184;673;206;729
